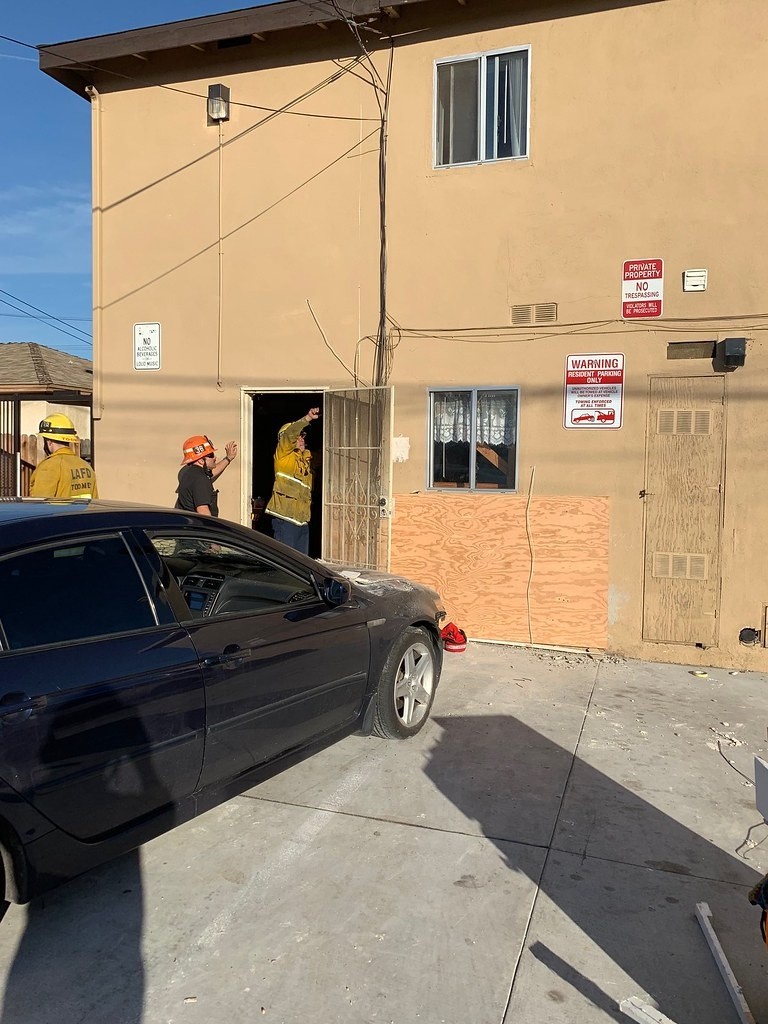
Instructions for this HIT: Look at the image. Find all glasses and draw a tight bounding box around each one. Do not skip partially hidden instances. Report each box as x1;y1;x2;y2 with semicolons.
203;452;214;458
299;431;306;438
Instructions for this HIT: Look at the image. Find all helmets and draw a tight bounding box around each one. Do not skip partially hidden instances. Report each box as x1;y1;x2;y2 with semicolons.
278;423;294;442
36;413;82;443
180;436;219;466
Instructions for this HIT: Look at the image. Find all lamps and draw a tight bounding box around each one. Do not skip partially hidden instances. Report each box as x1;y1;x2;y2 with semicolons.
207;84;231;122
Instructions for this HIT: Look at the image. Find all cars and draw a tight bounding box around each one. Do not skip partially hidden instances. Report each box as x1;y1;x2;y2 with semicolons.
2;500;443;906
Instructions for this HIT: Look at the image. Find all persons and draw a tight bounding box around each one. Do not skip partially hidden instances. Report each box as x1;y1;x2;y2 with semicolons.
174;435;237;518
29;412;99;499
265;407;322;555
